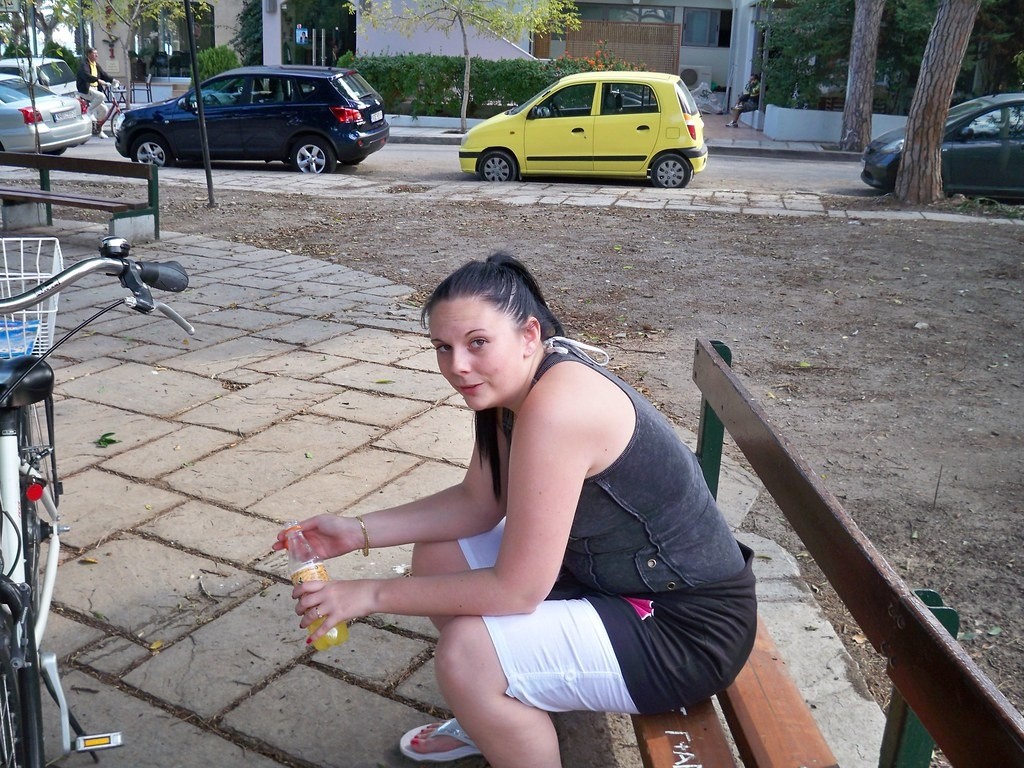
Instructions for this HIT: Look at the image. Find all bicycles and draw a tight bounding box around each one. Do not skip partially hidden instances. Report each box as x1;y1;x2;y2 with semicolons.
75;82;131;140
0;231;199;768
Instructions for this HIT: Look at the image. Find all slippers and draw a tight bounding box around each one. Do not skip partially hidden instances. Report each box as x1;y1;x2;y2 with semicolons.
400;717;483;761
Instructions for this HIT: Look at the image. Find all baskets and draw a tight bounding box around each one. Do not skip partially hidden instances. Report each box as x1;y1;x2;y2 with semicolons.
0;238;64;361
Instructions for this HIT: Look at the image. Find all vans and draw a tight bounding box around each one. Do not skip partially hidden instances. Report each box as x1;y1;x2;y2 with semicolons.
0;55;87;115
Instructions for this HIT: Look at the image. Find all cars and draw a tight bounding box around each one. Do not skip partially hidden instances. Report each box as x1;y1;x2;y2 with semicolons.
0;74;94;154
458;71;709;189
861;91;1024;210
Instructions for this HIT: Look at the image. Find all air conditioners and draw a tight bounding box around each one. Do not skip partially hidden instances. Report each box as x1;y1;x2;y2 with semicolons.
677;65;712;90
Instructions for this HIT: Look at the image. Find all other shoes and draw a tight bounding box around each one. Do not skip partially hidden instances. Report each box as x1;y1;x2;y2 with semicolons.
87;112;97;121
731;103;743;111
725;120;738;128
97;131;108;138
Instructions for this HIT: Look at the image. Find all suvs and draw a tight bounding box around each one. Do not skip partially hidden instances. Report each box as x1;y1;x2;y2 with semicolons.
112;61;392;176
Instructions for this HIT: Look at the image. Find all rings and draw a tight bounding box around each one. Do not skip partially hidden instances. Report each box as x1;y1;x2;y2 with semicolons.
315;606;323;619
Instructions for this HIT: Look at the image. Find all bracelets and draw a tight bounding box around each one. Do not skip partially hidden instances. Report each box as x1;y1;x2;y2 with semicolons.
354;516;369;557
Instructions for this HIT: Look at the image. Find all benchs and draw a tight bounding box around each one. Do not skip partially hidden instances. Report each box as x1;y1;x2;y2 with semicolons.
0;150;160;247
630;335;1023;768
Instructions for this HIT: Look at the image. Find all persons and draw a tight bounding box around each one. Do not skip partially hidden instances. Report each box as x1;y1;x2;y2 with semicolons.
326;43;338;67
272;254;758;768
725;74;761;128
77;48;120;139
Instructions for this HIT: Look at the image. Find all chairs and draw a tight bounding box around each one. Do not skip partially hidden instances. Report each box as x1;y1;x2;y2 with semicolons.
603;92;625;114
124;49;195;76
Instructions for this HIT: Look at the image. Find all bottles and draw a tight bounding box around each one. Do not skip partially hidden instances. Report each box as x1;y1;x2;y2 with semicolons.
282;520;350;652
